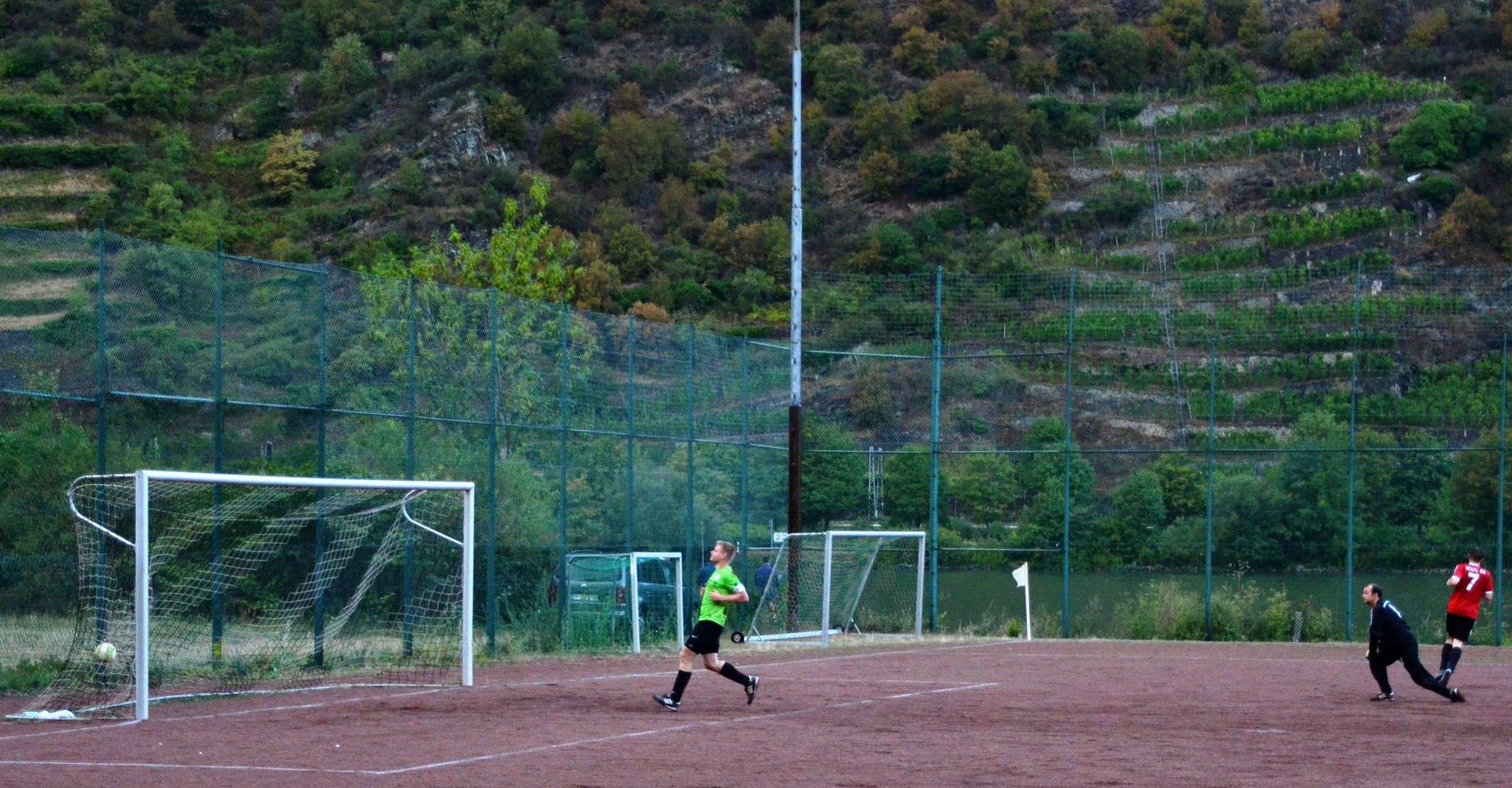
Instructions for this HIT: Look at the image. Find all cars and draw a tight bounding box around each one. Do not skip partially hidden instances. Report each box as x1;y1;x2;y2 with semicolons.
546;550;701;637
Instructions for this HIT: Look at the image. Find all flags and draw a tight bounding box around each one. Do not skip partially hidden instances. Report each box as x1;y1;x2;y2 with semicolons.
1011;561;1027;588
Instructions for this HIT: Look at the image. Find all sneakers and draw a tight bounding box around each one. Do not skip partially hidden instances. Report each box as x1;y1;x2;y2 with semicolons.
745;676;759;704
1434;669;1445;680
1370;691;1395;702
1439;668;1451;686
1450;688;1465;702
652;693;681;711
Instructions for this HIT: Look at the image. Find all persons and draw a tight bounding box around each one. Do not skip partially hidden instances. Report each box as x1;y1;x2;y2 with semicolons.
754;555;780;627
1361;583;1466;703
651;540;759;711
696;557;716;597
1434;547;1493;688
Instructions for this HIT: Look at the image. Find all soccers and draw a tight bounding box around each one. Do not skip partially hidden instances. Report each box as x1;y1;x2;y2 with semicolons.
95;642;116;662
